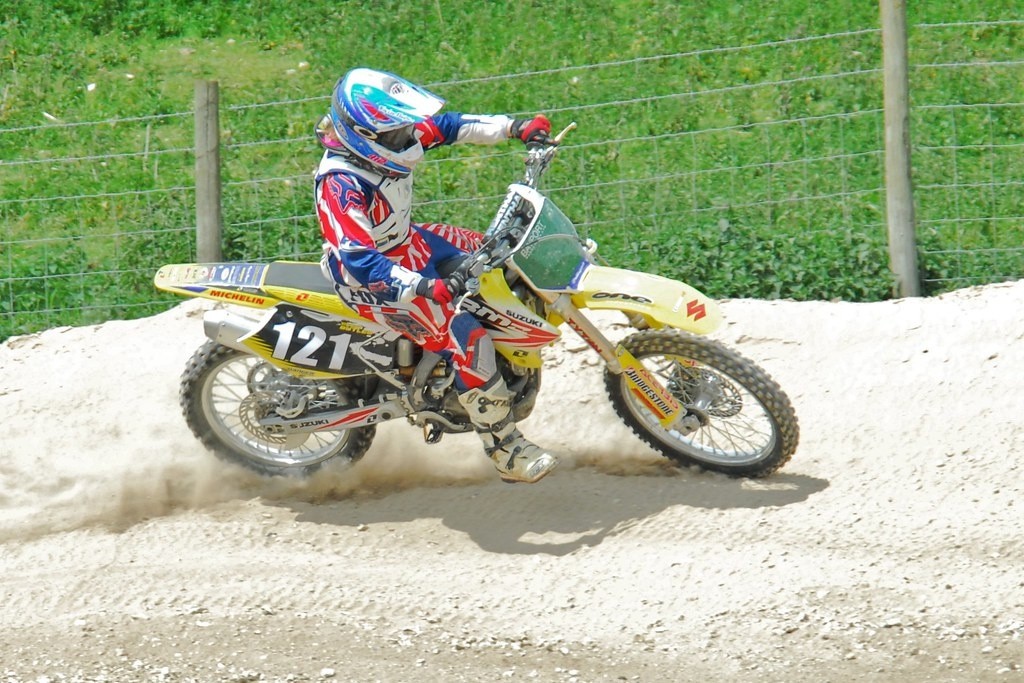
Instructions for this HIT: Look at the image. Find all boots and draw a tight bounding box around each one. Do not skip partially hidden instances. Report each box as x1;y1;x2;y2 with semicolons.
454;368;561;484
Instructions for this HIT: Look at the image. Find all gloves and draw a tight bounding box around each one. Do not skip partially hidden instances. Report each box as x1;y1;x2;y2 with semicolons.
416;276;462;304
508;113;552;145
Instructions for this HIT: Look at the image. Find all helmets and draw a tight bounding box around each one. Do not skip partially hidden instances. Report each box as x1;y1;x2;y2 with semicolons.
314;66;445;180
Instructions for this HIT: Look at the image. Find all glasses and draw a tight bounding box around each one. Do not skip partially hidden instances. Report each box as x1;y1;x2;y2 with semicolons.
373;123;418;149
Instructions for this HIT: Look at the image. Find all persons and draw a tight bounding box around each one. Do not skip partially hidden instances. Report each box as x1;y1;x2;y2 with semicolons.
314;67;559;483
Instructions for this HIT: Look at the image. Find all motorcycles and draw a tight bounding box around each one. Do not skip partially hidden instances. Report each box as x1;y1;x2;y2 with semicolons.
150;116;800;482
